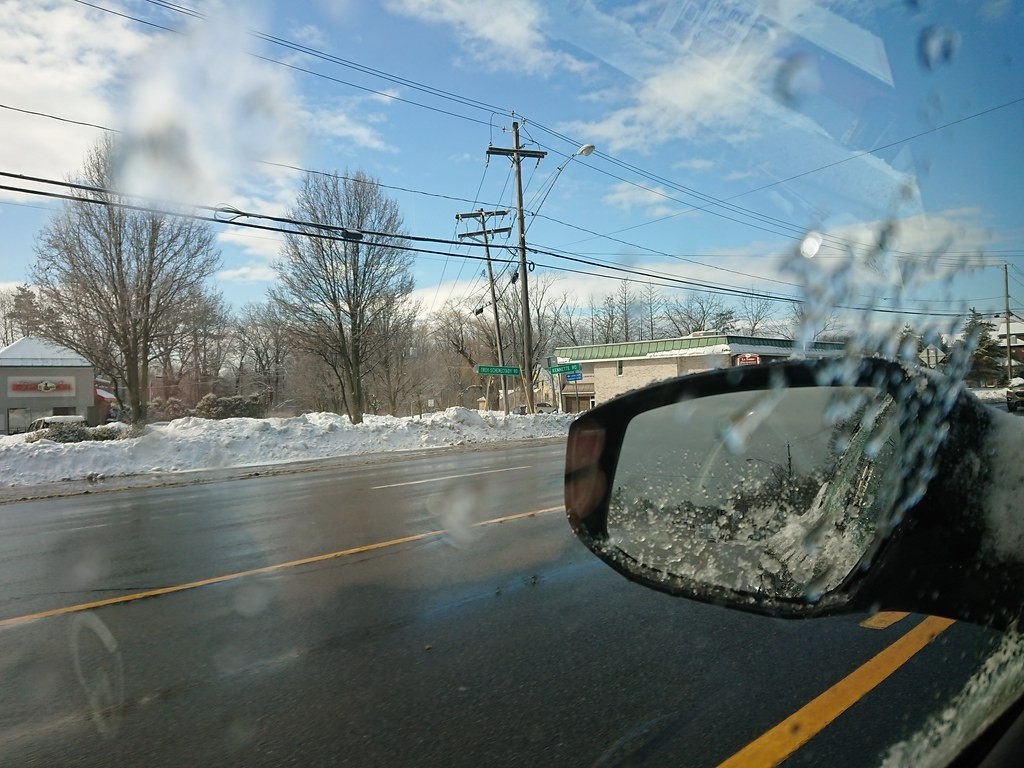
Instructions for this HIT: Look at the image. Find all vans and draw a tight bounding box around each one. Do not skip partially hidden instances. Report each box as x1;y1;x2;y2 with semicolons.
26;415;90;432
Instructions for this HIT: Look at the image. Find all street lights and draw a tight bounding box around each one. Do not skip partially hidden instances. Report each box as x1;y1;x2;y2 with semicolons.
519;144;596;415
743;456;792;521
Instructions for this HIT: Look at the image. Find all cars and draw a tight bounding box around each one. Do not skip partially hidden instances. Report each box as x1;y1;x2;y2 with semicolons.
514;405;527;413
1007;381;1024;412
533;403;557;414
